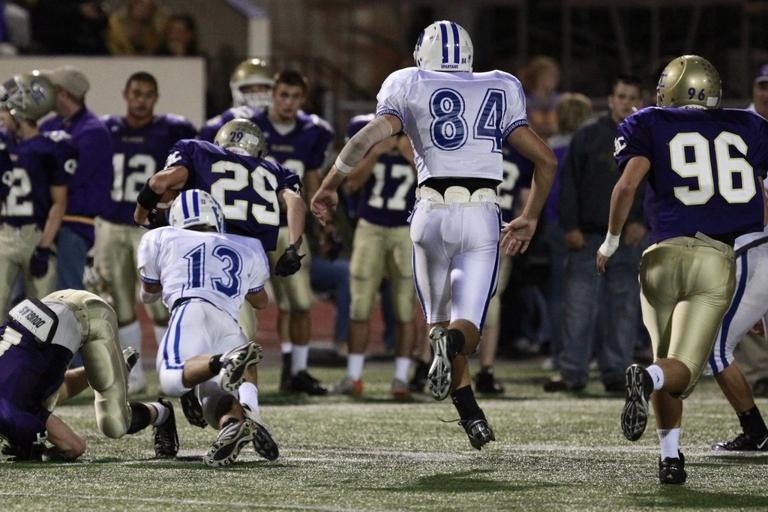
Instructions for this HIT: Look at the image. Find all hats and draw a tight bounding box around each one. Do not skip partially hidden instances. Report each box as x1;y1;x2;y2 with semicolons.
755;62;768;85
41;66;92;102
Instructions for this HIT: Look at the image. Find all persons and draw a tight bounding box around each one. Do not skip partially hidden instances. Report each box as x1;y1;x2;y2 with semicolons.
136;117;305;460
707;232;767;459
134;187;280;470
593;52;767;487
1;286;180;461
306;17;559;451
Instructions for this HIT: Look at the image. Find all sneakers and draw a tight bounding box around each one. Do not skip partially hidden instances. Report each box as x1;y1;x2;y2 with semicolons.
711;429;768;452
330;375;364;399
179;388;209;429
658;449;687;485
152;397;180;459
239;402;280;461
389;378;411;399
217;340;264;392
462;414;497;452
279;368;329;397
619;363;650;442
474;374;506;396
122;345;140;376
542;371;588;394
203;418;259;468
425;325;455;401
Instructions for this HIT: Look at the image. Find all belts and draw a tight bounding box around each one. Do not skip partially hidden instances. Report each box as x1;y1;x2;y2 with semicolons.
416;200;497;210
660;233;736;259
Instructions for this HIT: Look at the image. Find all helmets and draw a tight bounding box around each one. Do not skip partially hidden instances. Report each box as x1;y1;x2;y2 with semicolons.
412;18;475;73
1;70;57;123
212;117;270;161
655;53;725;111
228;57;276;113
168;188;226;234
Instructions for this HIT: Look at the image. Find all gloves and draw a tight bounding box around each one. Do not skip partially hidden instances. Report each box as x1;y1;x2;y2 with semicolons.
28;245;51;279
274;246;302;277
